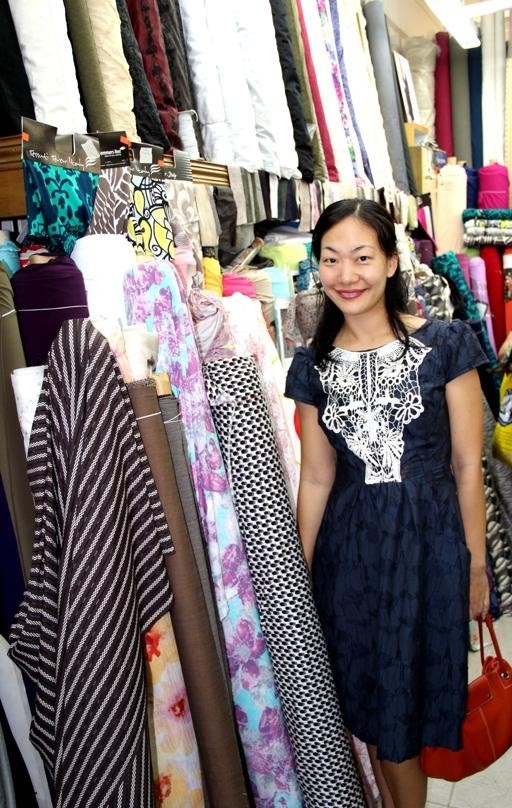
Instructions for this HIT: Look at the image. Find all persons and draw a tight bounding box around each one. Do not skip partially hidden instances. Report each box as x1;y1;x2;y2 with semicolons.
283;198;492;807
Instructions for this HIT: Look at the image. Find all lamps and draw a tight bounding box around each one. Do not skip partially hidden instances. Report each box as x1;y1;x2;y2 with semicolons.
422;1;482;51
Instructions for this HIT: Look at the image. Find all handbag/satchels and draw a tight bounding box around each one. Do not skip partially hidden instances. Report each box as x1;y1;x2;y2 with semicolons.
420;617;512;781
